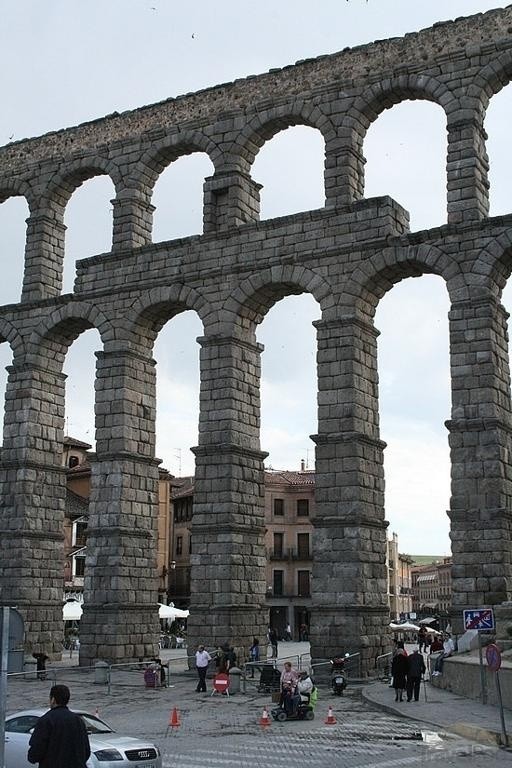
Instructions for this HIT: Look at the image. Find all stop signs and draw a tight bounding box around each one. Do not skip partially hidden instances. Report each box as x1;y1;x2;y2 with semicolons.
214;672;230;691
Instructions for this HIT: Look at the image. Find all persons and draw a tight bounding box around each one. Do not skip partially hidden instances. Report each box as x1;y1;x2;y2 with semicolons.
28;685;91;768
278;662;297;706
269;622;309;658
390;624;454;702
288;671;313;717
195;644;212;692
249;639;262;678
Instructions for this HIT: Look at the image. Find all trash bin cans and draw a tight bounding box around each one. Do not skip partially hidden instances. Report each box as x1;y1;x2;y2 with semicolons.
94;661;109;683
228;667;241;694
24;660;37;679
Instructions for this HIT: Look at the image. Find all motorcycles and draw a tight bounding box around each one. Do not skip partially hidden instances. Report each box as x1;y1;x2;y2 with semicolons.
329;652;353;696
271;670;316;720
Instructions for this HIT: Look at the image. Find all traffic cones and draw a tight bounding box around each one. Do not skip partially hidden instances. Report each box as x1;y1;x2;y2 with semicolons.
168;706;180;726
95;707;99;718
324;705;338;723
259;705;270;724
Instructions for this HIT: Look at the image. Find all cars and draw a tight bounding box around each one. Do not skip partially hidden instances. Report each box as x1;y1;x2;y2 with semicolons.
5;707;162;767
